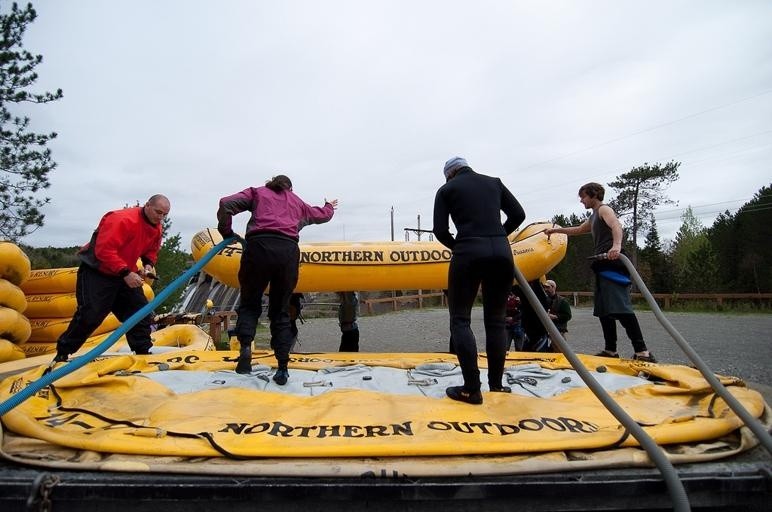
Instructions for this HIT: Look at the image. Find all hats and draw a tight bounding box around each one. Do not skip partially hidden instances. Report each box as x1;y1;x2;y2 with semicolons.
544;279;556;289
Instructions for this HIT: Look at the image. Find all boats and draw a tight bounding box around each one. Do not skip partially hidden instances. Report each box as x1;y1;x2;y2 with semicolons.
76;324;216;354
0;338;26;363
28;312;122;342
120;324;216;354
0;279;28;313
0;306;32;346
24;281;155;319
20;256;157;294
19;329;127;358
0;242;31;288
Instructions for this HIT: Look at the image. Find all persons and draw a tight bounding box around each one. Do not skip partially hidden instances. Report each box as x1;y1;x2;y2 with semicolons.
432;156;526;406
511;279;548;354
544;182;657;363
544;281;572;354
502;291;524;352
285;293;305;353
217;174;339;386
338;292;360;353
51;193;170;363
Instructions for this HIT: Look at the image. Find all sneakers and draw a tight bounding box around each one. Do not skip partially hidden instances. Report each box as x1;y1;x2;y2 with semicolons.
446;385;483;405
272;370;289;386
631;352;656;363
594;351;619;358
490;387;511;393
234;354;252;375
